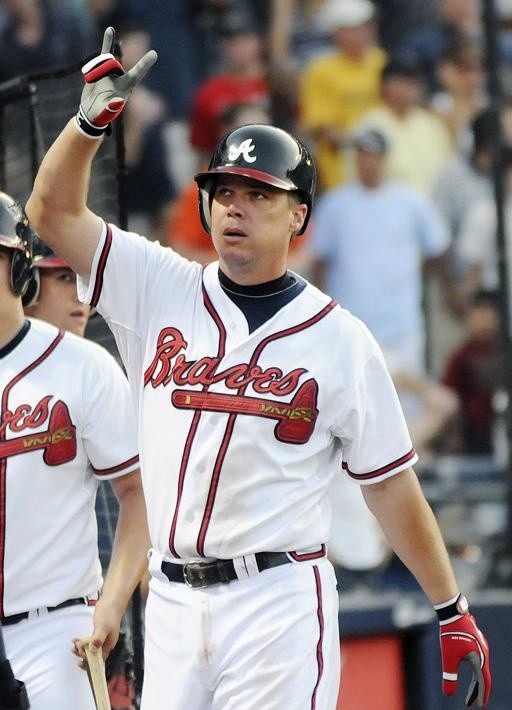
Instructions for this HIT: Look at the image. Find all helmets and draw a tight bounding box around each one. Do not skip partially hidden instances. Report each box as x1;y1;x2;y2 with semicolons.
0;190;31;296
192;122;318;237
22;222;70;308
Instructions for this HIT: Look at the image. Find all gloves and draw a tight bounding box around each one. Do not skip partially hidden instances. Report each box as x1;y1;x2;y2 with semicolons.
72;26;158;140
432;591;492;710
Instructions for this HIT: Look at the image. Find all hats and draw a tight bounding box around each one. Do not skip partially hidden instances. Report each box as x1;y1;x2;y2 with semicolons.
352;129;386;157
315;0;374;31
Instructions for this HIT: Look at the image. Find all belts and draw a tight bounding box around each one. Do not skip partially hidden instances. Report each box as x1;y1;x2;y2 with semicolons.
0;596;87;627
161;551;293;591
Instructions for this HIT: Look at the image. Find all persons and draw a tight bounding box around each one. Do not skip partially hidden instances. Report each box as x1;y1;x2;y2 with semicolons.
21;211;154;709
0;183;155;710
19;23;498;710
